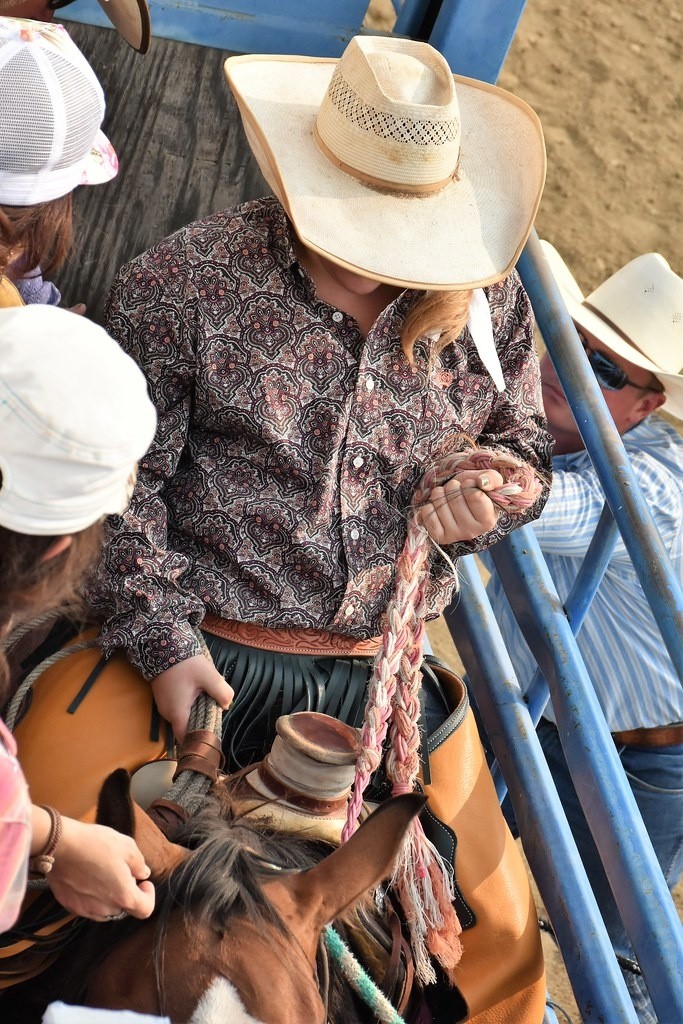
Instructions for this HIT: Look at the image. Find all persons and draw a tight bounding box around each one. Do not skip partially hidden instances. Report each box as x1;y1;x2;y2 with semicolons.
85;32;554;1023
0;0;151;315
475;237;683;1024
0;302;156;937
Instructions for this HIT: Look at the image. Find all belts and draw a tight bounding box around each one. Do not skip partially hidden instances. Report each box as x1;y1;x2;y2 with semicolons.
197;610;388;656
612;724;683;748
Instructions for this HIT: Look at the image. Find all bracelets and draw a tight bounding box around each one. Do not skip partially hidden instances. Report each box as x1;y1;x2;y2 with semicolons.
30;804;61;874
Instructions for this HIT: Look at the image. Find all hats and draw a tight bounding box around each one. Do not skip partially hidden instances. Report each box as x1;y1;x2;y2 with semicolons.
0;15;119;206
98;0;151;55
0;304;159;535
538;239;683;422
226;35;548;292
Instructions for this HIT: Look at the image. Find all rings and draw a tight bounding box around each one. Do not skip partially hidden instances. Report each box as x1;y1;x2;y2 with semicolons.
105;910;128;921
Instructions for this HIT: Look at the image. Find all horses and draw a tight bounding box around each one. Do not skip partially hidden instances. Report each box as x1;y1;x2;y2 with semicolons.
0;768;429;1024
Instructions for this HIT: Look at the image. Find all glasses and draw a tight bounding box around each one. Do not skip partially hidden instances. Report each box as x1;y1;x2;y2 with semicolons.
577;329;662;394
47;0;75;9
